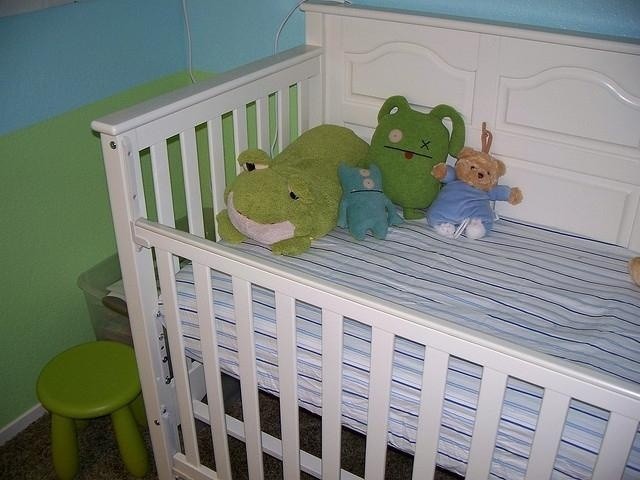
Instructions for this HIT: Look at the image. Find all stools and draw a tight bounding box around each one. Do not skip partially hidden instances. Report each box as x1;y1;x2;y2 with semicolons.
35;342;151;479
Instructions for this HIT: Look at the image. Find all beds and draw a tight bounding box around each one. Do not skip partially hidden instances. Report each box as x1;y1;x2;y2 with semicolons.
90;1;638;478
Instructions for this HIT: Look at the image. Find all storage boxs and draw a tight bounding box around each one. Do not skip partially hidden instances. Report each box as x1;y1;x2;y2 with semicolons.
72;207;216;353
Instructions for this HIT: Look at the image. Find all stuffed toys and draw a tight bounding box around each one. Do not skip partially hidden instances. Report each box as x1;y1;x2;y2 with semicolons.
429;120;522;241
369;96;464;218
216;125;369;255
336;159;402;242
